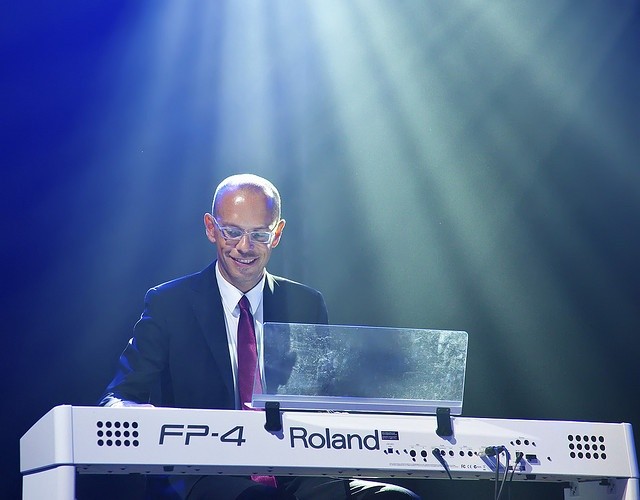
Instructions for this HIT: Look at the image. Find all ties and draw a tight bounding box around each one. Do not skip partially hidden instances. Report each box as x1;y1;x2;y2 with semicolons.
238;295;277;488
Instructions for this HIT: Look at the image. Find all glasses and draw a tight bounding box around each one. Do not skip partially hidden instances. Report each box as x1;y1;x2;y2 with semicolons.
212;216;279;243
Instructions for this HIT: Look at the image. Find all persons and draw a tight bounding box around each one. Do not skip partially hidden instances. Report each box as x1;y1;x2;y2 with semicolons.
99;174;421;500
